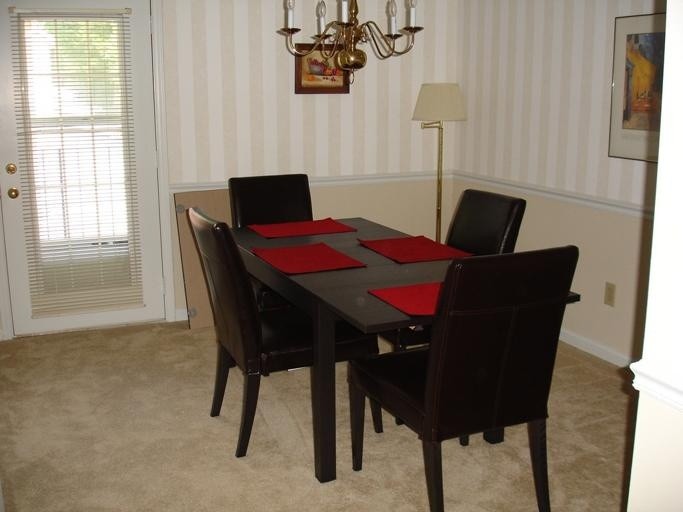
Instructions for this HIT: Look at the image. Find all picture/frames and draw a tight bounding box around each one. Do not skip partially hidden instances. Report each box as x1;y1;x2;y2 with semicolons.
295;44;349;94
608;13;666;164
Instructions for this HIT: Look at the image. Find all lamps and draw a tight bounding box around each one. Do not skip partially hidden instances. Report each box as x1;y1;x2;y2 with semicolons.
281;0;424;83
412;83;468;244
